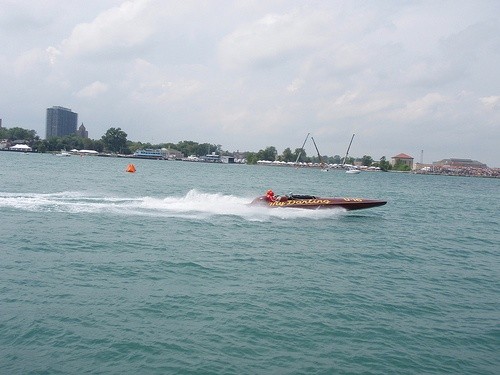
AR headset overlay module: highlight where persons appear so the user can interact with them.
[267,190,276,202]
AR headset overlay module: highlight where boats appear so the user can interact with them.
[131,147,171,161]
[246,193,387,213]
[345,168,362,175]
